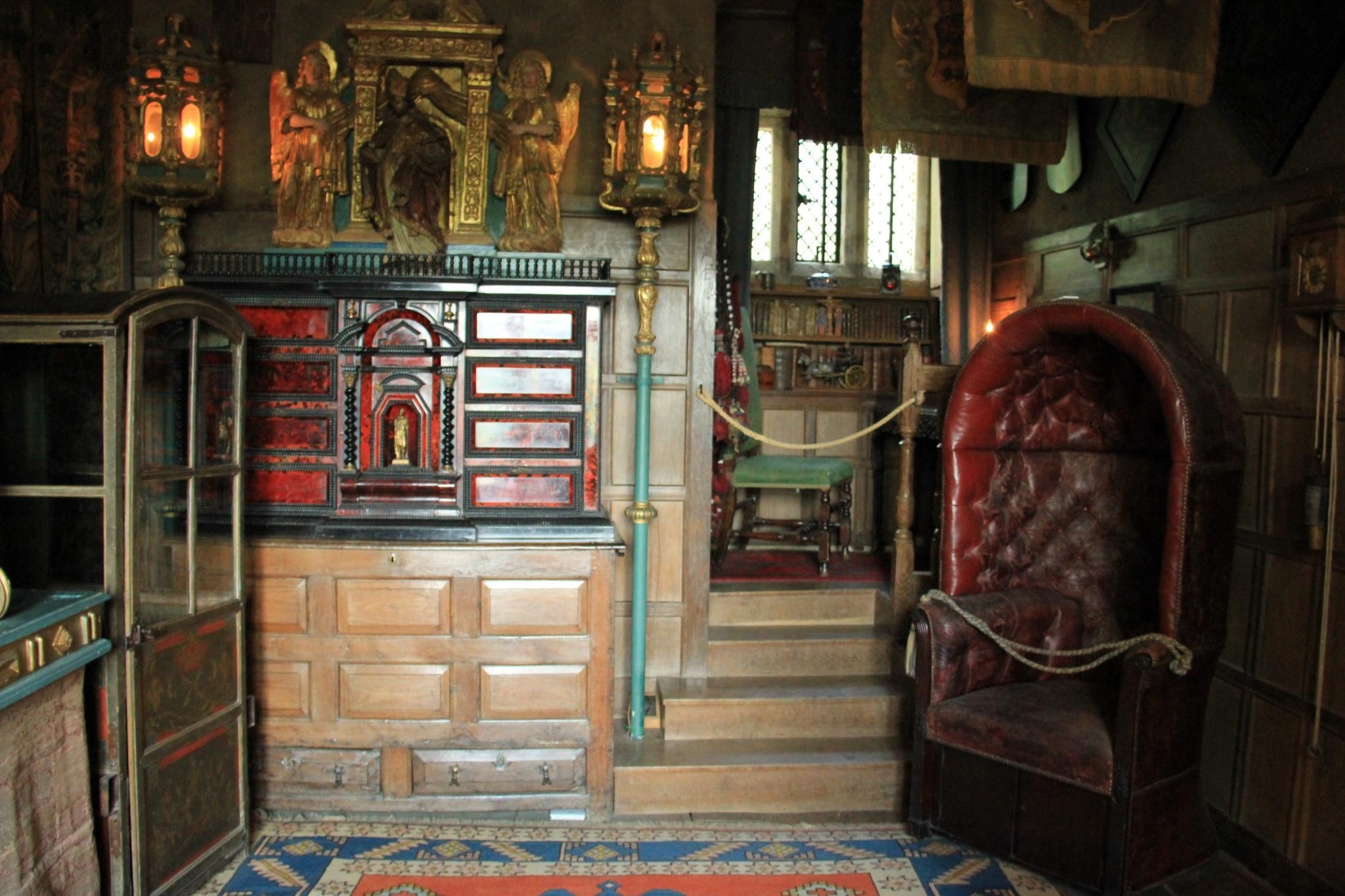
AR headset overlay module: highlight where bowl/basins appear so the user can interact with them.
[808,269,837,286]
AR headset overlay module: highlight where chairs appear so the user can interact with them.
[715,307,854,577]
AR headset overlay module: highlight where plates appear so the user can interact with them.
[806,286,839,293]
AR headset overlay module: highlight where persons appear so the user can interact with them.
[391,408,411,464]
[269,50,349,249]
[215,404,234,454]
[358,68,452,275]
[493,58,563,252]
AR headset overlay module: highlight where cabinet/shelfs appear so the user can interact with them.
[748,284,941,412]
[0,287,255,896]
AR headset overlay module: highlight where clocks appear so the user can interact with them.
[1277,199,1345,321]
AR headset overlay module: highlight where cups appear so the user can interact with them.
[754,270,776,292]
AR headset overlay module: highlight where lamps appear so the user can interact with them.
[599,22,710,221]
[122,9,225,288]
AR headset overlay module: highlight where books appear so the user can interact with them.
[750,298,930,340]
[752,342,936,392]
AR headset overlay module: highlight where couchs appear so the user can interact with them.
[906,300,1238,896]
